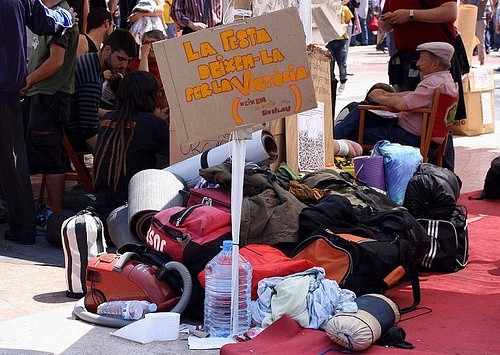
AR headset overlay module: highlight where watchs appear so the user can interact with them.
[410,10,414,21]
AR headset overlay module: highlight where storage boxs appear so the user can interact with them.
[454,65,495,136]
[264,44,334,176]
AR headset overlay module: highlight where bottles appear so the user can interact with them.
[97,300,157,320]
[380,265,406,295]
[203,240,252,338]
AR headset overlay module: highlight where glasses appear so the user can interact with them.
[104,20,117,30]
[142,41,155,45]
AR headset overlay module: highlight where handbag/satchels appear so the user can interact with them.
[46,139,470,350]
[368,14,379,31]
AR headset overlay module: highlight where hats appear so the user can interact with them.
[415,42,455,61]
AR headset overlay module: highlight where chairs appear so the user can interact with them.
[38,130,93,204]
[358,83,459,166]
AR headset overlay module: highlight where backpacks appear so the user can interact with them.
[481,156,500,199]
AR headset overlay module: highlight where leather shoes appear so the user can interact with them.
[4,230,36,245]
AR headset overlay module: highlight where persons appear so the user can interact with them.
[0,0,79,246]
[94,70,169,202]
[460,0,500,66]
[68,0,177,150]
[19,0,79,235]
[331,0,395,83]
[128,29,168,111]
[379,0,470,174]
[171,0,220,35]
[333,42,457,145]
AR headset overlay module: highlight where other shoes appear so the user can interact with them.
[473,51,478,56]
[486,50,490,54]
[339,83,345,92]
[493,47,499,51]
[376,43,384,50]
